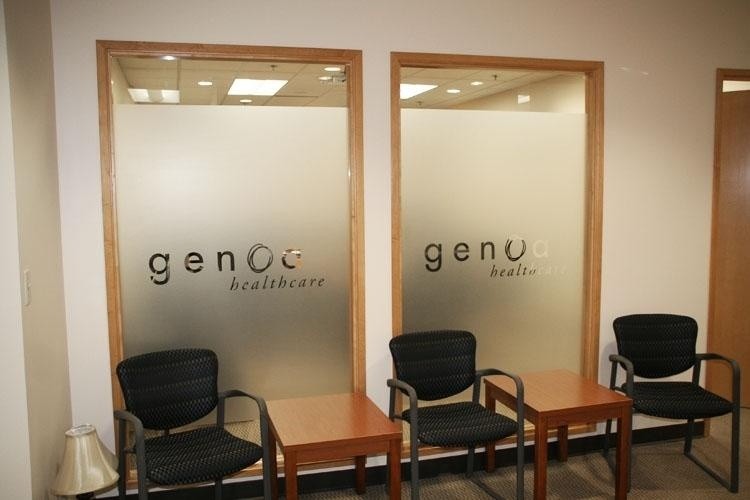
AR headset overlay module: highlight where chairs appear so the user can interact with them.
[386,328,526,500]
[114,345,273,497]
[605,314,742,496]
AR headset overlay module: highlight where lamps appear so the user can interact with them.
[50,424,121,498]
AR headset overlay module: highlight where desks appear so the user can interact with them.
[481,365,632,499]
[264,389,403,499]
